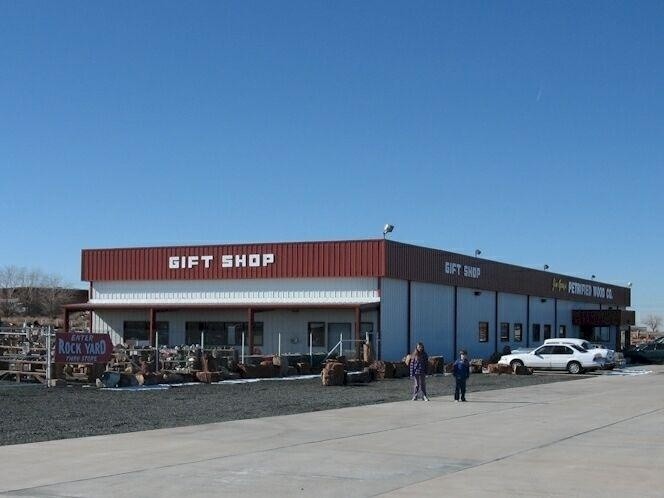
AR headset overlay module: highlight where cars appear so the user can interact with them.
[496,343,603,376]
[510,338,616,371]
[618,336,664,364]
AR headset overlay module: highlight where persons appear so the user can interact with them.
[409,342,429,402]
[451,348,470,403]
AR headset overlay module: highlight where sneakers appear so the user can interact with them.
[423,395,429,401]
[412,395,417,400]
[455,399,465,402]
